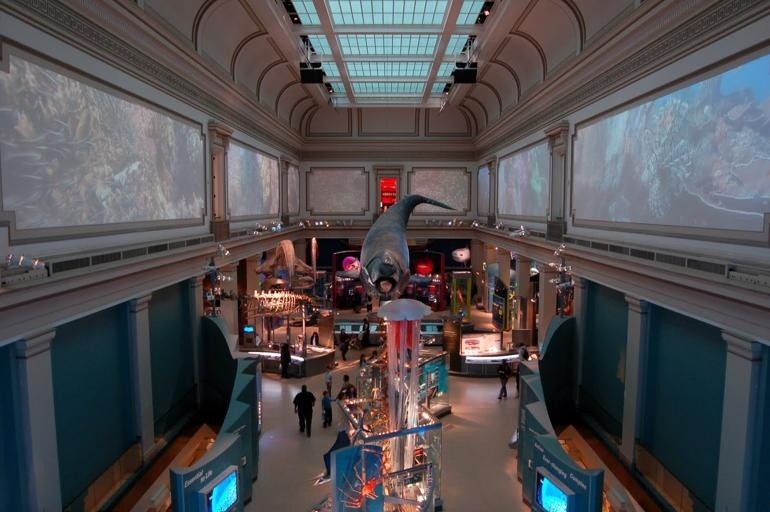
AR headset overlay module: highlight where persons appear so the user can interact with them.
[325,366,332,396]
[338,329,350,360]
[280,343,292,379]
[359,353,367,368]
[320,391,336,428]
[326,285,332,298]
[292,384,316,437]
[370,351,377,360]
[351,291,372,313]
[362,318,371,347]
[310,332,319,345]
[335,374,357,410]
[515,343,528,398]
[472,283,478,306]
[497,357,511,399]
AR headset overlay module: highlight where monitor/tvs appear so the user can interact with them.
[243,326,256,333]
[534,465,576,512]
[197,464,241,512]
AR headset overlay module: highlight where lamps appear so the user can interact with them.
[453,41,478,84]
[7,254,45,270]
[300,41,323,83]
[448,217,575,293]
[203,218,329,280]
